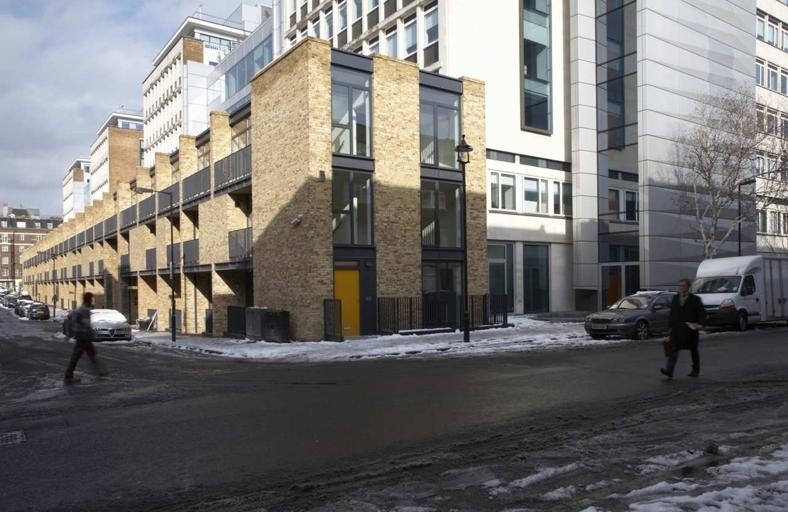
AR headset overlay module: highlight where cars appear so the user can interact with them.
[584,290,679,340]
[1,285,51,320]
[63,308,131,341]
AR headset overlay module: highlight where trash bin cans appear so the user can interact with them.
[246,307,268,340]
[264,311,289,342]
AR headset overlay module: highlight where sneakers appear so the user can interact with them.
[64,378,81,386]
[688,369,700,377]
[660,367,673,378]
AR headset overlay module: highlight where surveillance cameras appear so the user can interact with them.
[290,215,303,225]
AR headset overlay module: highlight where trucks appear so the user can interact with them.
[691,254,788,333]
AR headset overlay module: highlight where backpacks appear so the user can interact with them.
[63,311,80,339]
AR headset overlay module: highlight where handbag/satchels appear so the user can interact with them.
[663,341,670,358]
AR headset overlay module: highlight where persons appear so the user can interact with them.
[62,292,108,384]
[661,278,706,377]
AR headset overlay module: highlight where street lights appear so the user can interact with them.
[10,262,22,297]
[454,135,475,343]
[134,186,176,341]
[36,250,58,317]
[738,177,758,256]
